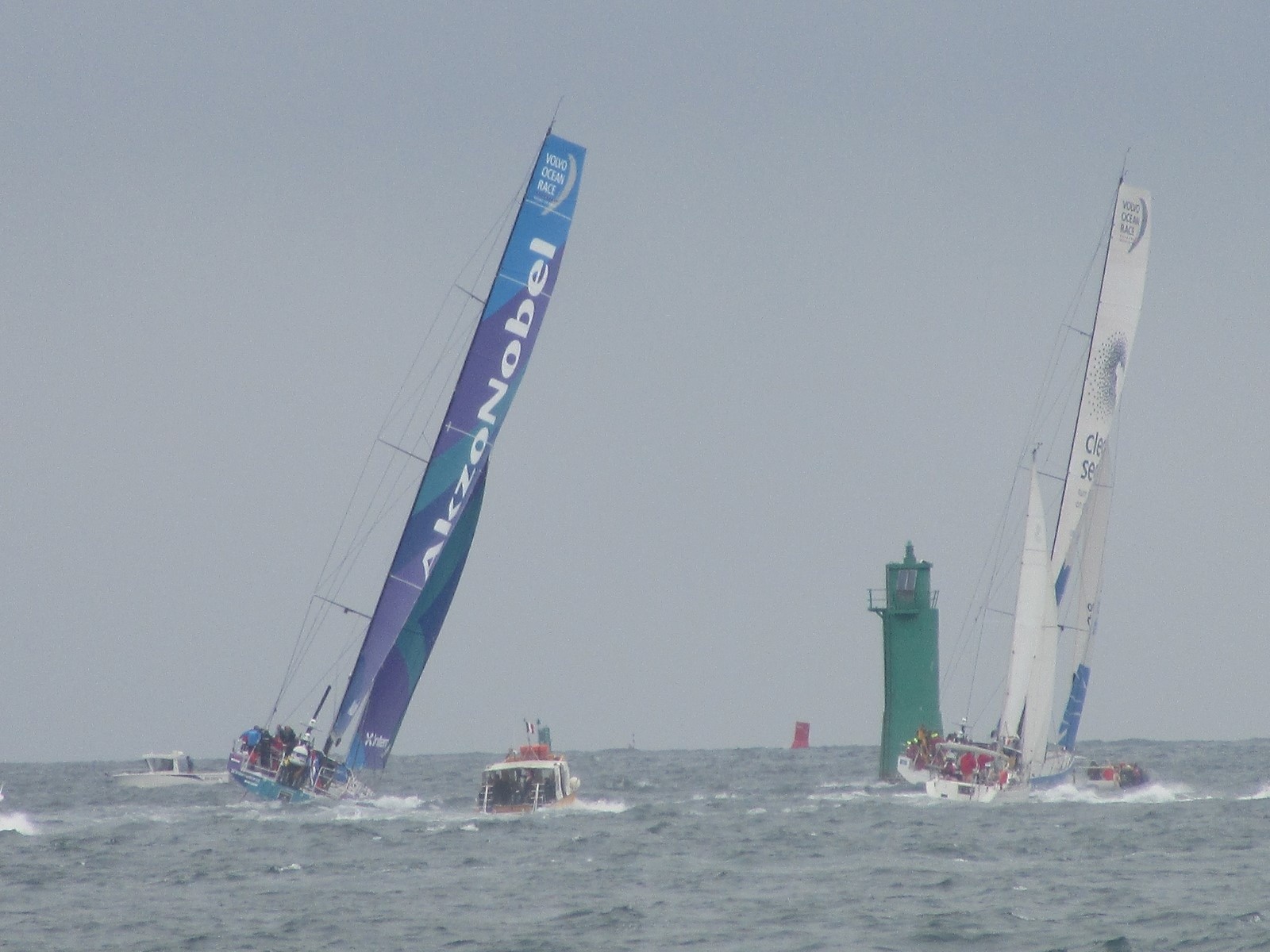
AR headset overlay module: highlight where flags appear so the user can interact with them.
[525,722,535,734]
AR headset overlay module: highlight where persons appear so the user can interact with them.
[237,725,308,789]
[906,724,993,784]
[490,769,533,806]
[1088,759,1145,788]
[185,756,194,774]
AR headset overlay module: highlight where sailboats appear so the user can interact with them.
[227,93,587,804]
[896,148,1151,792]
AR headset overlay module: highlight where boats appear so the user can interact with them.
[109,750,230,791]
[473,718,581,814]
[926,772,1018,804]
